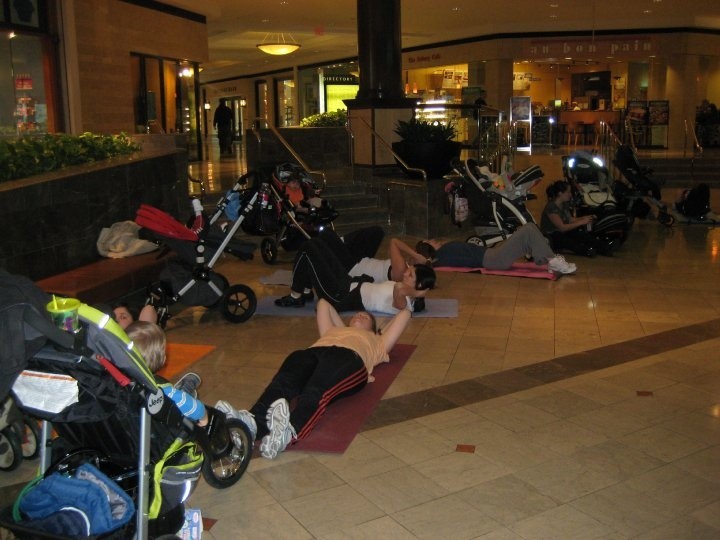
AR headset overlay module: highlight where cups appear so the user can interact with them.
[47,294,83,334]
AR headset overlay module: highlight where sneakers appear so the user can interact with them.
[548,258,576,274]
[260,397,294,458]
[217,400,257,444]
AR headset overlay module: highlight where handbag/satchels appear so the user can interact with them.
[447,193,468,222]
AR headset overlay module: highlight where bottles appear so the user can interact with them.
[257,182,271,210]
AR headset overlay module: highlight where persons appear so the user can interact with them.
[473,90,488,120]
[273,235,437,316]
[111,305,159,331]
[416,221,577,274]
[676,183,711,220]
[696,100,719,125]
[124,321,210,432]
[212,97,234,156]
[317,227,433,286]
[540,179,596,256]
[214,298,411,459]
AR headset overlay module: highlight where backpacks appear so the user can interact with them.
[675,183,712,219]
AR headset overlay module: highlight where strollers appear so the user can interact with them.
[244,162,340,262]
[608,144,675,231]
[1,274,256,539]
[563,152,635,263]
[132,171,281,333]
[454,160,543,257]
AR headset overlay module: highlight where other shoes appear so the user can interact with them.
[174,374,202,397]
[274,291,314,307]
